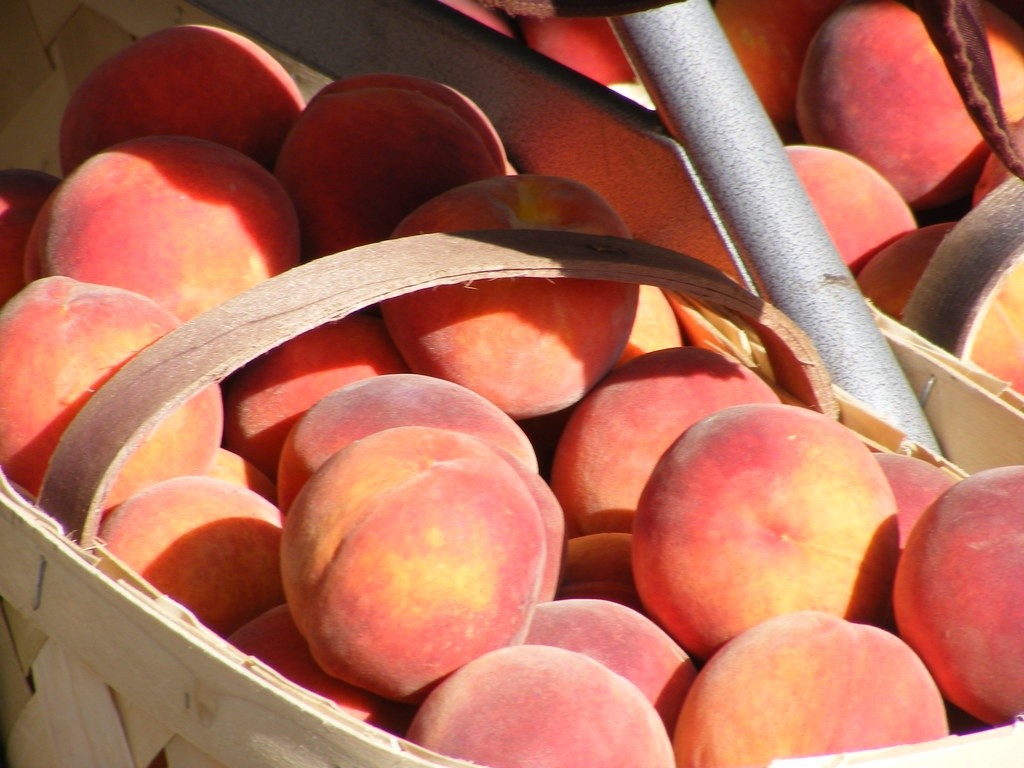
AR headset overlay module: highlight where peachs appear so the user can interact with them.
[0,0,1024,768]
